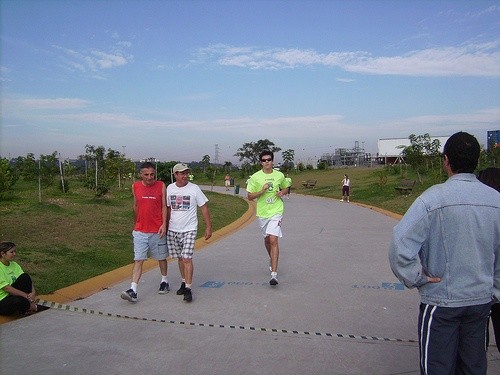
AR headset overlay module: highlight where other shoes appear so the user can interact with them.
[183,288,192,301]
[345,200,349,202]
[34,304,50,313]
[177,282,185,295]
[340,199,343,201]
[269,272,278,286]
[269,259,272,271]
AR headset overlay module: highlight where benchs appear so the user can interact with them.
[302,178,317,189]
[394,179,415,193]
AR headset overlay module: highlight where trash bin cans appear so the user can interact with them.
[234,184,240,196]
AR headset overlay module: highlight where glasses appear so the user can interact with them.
[262,158,272,162]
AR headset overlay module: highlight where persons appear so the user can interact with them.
[247,150,289,286]
[121,162,170,303]
[167,163,212,302]
[246,175,251,199]
[283,173,292,199]
[0,241,37,316]
[188,171,194,183]
[388,131,500,375]
[224,173,232,191]
[341,173,351,203]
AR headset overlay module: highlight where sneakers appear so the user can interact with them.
[120,289,137,302]
[158,281,169,293]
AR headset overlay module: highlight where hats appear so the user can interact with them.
[173,163,192,173]
